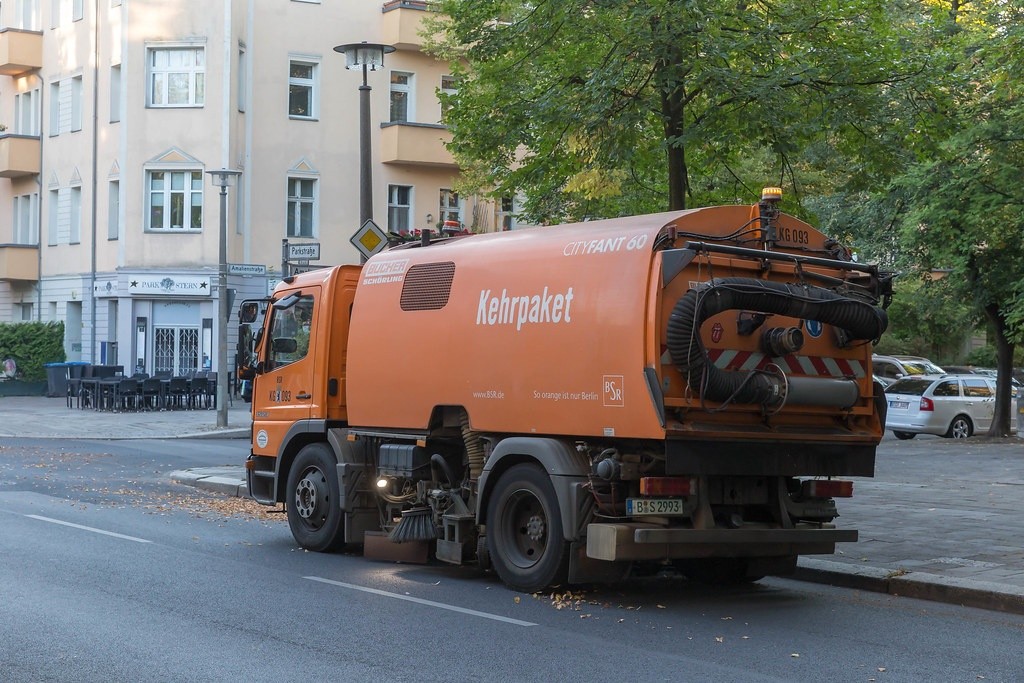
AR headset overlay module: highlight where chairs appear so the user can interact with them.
[67,366,233,413]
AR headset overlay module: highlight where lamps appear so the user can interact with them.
[426,214,432,225]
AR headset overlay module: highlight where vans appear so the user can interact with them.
[884,374,1024,440]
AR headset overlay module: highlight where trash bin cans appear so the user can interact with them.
[43,360,90,397]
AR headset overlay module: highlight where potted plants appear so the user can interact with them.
[0,124,9,134]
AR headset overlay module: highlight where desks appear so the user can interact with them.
[81,380,102,411]
[99,381,192,413]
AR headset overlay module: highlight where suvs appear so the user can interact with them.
[872,355,947,395]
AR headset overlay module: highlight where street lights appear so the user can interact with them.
[205,167,243,427]
[333,41,396,264]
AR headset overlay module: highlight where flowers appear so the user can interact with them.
[390,229,477,240]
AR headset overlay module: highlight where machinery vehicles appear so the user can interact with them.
[237,187,899,596]
